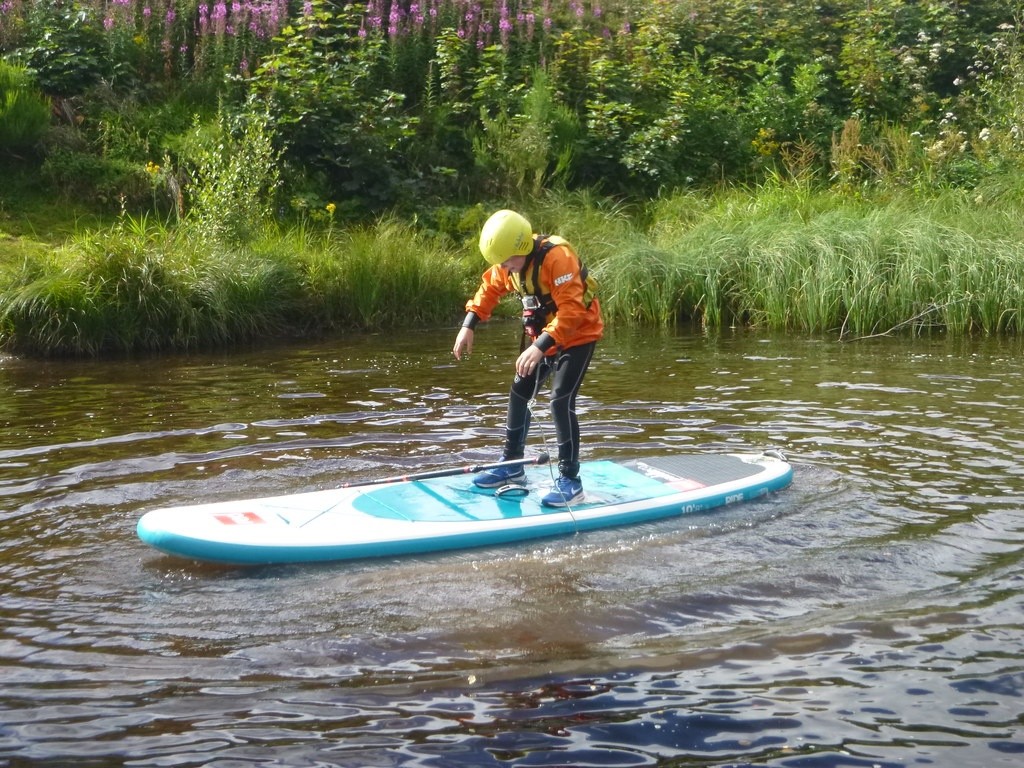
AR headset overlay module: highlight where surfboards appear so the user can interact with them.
[138,452,791,565]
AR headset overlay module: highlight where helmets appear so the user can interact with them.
[478,210,534,265]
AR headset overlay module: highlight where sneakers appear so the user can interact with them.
[541,475,585,507]
[472,457,527,488]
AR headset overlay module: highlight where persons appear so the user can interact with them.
[453,209,604,509]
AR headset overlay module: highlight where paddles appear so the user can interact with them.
[336,452,550,488]
